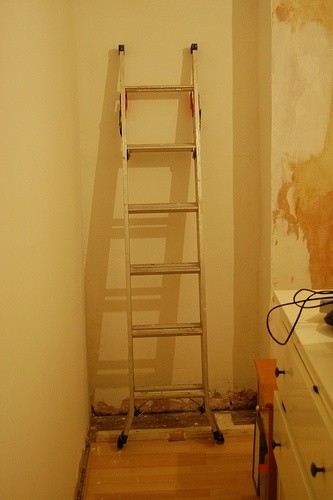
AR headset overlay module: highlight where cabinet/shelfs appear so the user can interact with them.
[270,289,333,500]
[251,356,279,500]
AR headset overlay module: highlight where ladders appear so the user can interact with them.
[117,42,224,447]
